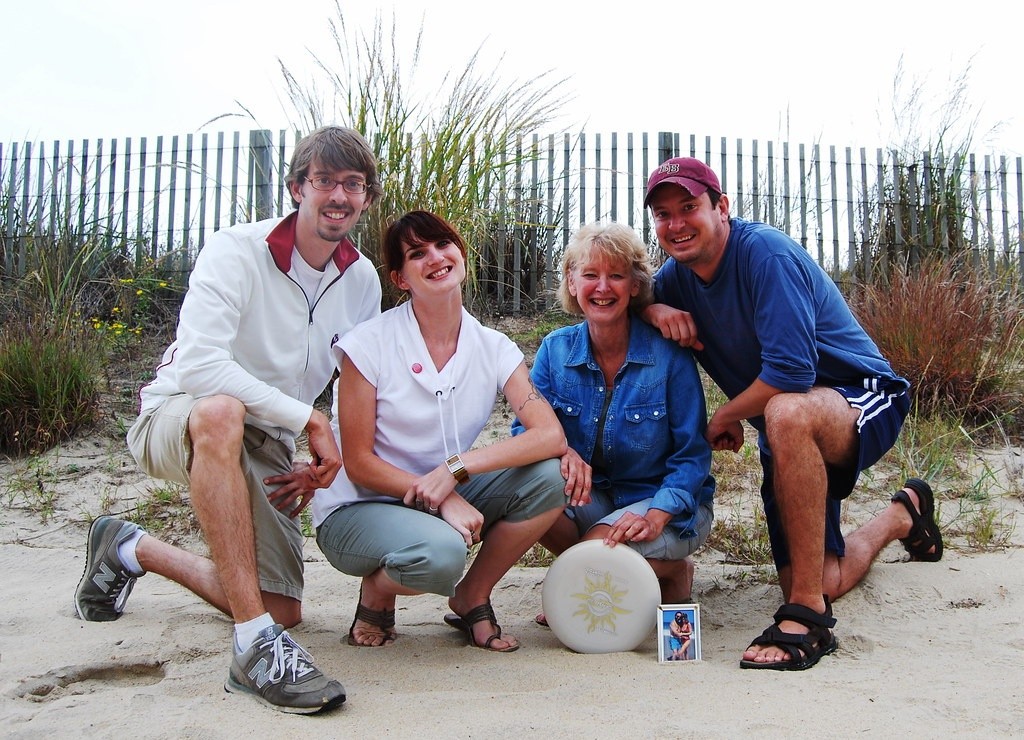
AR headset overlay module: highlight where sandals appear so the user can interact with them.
[740,594,838,671]
[890,478,943,563]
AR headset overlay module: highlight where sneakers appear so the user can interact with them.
[74,515,146,622]
[225,624,347,715]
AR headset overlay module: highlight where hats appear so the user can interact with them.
[643,156,721,210]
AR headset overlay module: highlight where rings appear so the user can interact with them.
[429,507,438,512]
[471,530,475,537]
[415,499,423,506]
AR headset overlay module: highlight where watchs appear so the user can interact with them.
[445,454,470,486]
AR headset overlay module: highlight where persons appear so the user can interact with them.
[75,125,384,714]
[311,211,572,653]
[628,158,944,670]
[511,221,716,627]
[669,611,692,661]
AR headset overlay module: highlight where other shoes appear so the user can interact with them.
[535,611,549,626]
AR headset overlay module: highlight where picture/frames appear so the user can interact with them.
[657,604,701,664]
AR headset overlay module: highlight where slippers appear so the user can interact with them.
[347,583,396,646]
[444,603,519,652]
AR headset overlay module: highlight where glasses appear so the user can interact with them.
[302,174,372,195]
